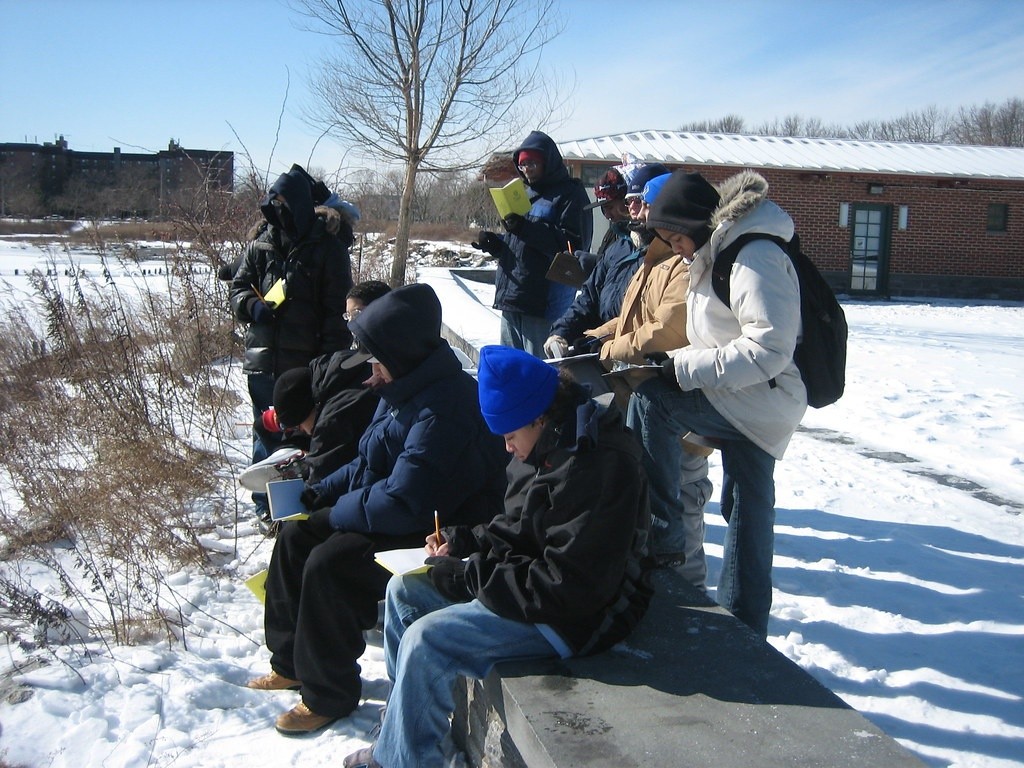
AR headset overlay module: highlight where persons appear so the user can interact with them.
[623,167,806,643]
[568,164,714,597]
[343,343,651,768]
[540,162,669,411]
[472,128,596,361]
[223,164,391,537]
[246,284,507,735]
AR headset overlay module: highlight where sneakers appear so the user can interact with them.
[275,702,338,735]
[248,670,303,689]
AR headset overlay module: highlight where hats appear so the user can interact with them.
[273,367,316,427]
[625,163,670,198]
[518,150,544,165]
[477,345,557,434]
[641,173,672,205]
[340,340,373,369]
[582,169,626,210]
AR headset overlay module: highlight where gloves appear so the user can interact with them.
[308,506,332,539]
[501,213,525,236]
[542,332,602,359]
[253,300,276,322]
[301,489,325,511]
[643,352,668,366]
[574,249,596,270]
[471,231,498,254]
[658,359,673,379]
[425,556,473,605]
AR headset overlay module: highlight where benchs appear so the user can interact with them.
[447,568,930,768]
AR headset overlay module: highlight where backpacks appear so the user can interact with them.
[712,233,847,409]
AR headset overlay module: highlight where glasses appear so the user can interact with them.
[270,198,289,209]
[294,424,300,432]
[518,162,538,171]
[624,198,643,207]
[342,308,362,321]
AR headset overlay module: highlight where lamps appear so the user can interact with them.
[838,200,849,231]
[897,202,910,232]
[868,184,885,195]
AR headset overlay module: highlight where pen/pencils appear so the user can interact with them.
[568,241,572,254]
[435,511,441,546]
[585,332,614,345]
[251,283,275,318]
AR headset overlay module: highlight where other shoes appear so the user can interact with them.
[646,550,688,569]
[342,746,382,768]
[257,512,274,536]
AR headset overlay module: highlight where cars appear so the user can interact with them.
[43,214,64,220]
[79,215,147,223]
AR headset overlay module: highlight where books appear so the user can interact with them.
[264,278,288,309]
[488,179,533,219]
[373,544,473,579]
[245,568,268,606]
[264,480,311,521]
